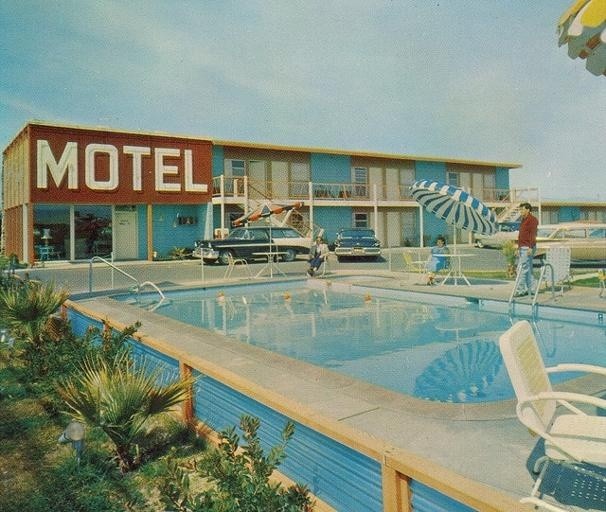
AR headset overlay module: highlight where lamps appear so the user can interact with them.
[40,228,52,246]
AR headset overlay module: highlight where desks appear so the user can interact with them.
[251,251,289,279]
[36,247,54,260]
[431,251,475,291]
[433,309,480,332]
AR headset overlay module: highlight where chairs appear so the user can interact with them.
[306,241,329,283]
[493,319,605,511]
[539,245,571,296]
[400,247,433,289]
[224,249,254,281]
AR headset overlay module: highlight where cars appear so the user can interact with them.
[334,227,381,260]
[473,221,520,250]
[527,222,606,267]
[193,226,311,265]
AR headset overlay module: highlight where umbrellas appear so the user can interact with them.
[408,178,498,286]
[232,202,304,279]
[412,331,505,402]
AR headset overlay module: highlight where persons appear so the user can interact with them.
[216,229,221,238]
[307,236,329,276]
[514,202,539,297]
[428,237,448,283]
